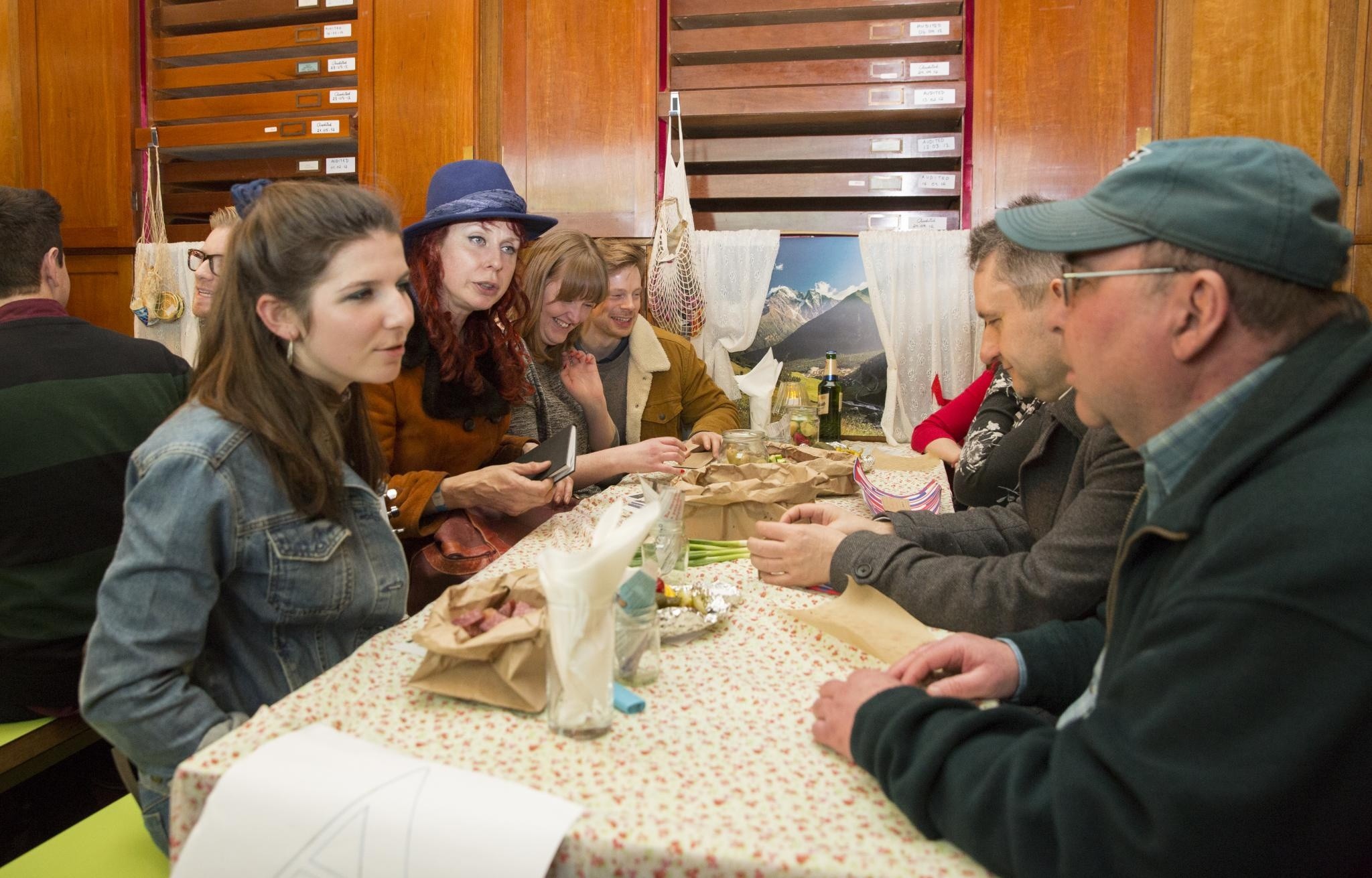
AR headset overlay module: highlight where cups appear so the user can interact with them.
[748,397,772,431]
[543,592,618,740]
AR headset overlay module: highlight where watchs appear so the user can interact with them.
[432,486,445,512]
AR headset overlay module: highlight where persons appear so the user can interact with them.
[186,205,243,318]
[0,189,197,725]
[576,237,738,489]
[516,230,690,499]
[77,177,416,862]
[746,195,1144,638]
[817,136,1372,878]
[358,160,574,618]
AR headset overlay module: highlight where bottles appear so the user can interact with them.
[615,602,661,685]
[641,519,689,584]
[785,406,819,447]
[818,350,842,443]
[718,429,769,465]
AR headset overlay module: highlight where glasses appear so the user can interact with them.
[1061,262,1233,307]
[188,247,226,279]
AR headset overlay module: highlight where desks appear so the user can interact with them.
[167,433,1006,878]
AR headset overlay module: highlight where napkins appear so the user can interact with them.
[525,493,664,732]
[732,346,784,431]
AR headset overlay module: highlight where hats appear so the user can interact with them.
[401,160,558,249]
[995,136,1353,290]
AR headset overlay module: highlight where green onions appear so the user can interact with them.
[628,539,753,566]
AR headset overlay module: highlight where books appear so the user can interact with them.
[514,424,577,484]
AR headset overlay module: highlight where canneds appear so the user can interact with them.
[784,406,819,447]
[718,428,769,464]
